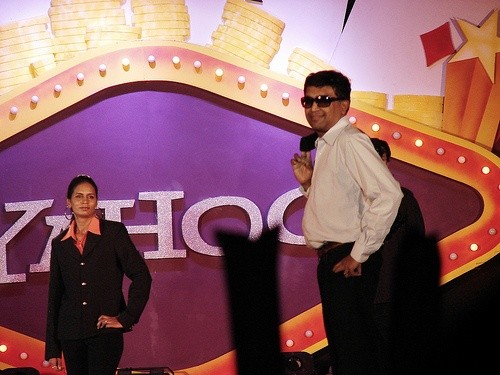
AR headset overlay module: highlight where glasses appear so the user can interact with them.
[301,95,344,108]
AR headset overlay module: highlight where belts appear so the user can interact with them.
[317,242,344,256]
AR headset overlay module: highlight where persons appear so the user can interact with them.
[44,175,151,375]
[290,69,404,360]
[370,137,426,311]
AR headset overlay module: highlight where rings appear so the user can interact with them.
[54,365,57,367]
[52,367,54,368]
[104,320,108,324]
